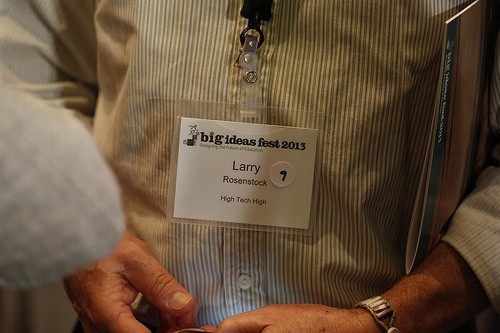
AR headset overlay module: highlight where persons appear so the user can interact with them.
[0,1,499,333]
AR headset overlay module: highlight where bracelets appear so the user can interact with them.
[353,295,400,333]
